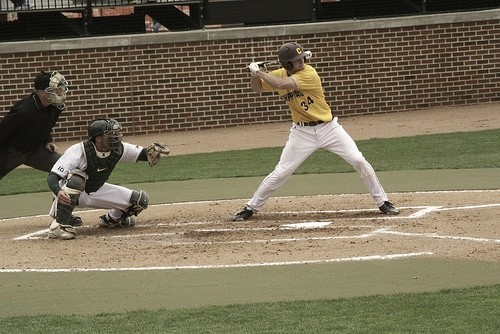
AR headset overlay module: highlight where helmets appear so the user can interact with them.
[278,41,308,70]
[34,70,67,90]
[86,118,119,141]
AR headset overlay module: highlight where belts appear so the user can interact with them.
[296,120,324,126]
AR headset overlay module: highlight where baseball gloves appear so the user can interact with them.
[146,141,170,167]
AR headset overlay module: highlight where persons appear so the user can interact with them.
[233,43,400,222]
[0,70,84,226]
[47,118,171,238]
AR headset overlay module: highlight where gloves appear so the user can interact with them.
[248,62,268,74]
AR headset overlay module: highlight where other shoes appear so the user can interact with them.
[69,214,83,225]
[48,223,77,240]
[233,207,253,221]
[98,212,136,228]
[380,201,399,215]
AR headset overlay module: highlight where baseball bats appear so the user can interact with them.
[246,51,312,73]
